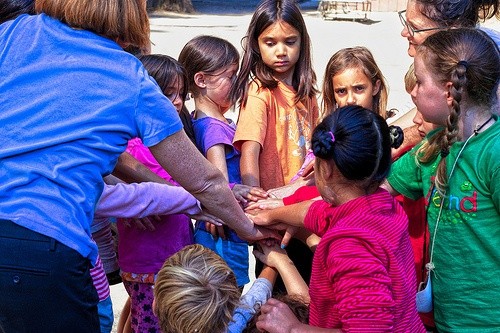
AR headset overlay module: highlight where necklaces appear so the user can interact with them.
[425,114,498,270]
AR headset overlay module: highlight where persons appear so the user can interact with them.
[244,105,429,333]
[152,244,279,333]
[86,0,500,333]
[242,243,311,333]
[265,28,500,333]
[0,0,288,333]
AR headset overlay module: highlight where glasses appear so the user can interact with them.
[398,10,442,37]
